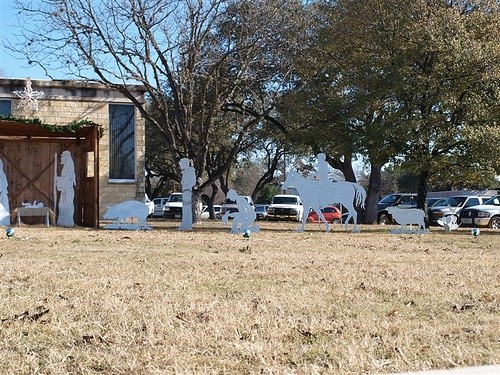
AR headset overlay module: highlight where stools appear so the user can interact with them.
[11,207,55,227]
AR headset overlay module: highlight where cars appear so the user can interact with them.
[163,192,183,219]
[377,193,418,225]
[256,206,269,220]
[306,206,342,224]
[148,198,169,219]
[201,206,222,219]
[459,195,500,229]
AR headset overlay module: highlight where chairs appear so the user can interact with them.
[173,197,179,202]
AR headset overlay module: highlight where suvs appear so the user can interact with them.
[267,194,304,223]
[428,196,491,226]
